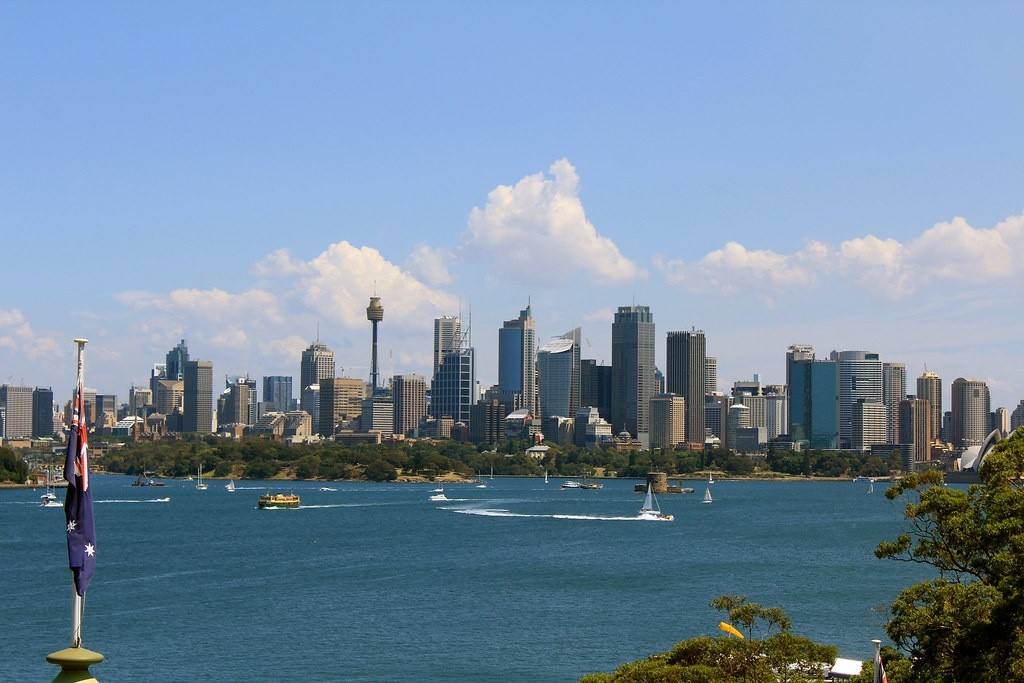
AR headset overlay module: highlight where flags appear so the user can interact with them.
[64,381,97,596]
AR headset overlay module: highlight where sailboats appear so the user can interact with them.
[489,466,493,480]
[702,487,713,503]
[132,460,165,486]
[709,469,714,483]
[41,447,57,500]
[544,470,549,483]
[224,478,235,492]
[869,483,874,493]
[637,482,674,521]
[196,464,208,490]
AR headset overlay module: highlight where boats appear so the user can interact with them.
[428,488,443,493]
[580,483,603,489]
[319,487,337,491]
[157,498,170,501]
[562,481,581,488]
[855,475,874,483]
[180,477,193,482]
[477,485,486,488]
[258,485,301,508]
[428,494,448,501]
[40,498,64,508]
